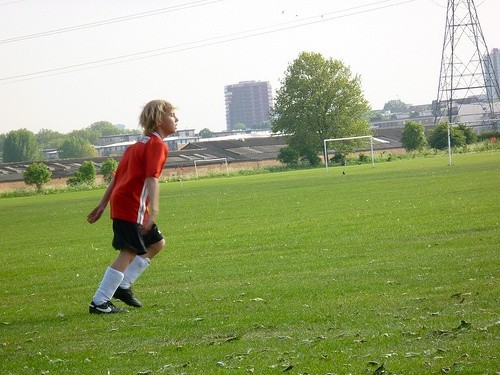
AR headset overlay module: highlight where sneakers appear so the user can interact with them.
[89,302,130,314]
[114,286,142,307]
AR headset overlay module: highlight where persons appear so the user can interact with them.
[87,99,180,314]
